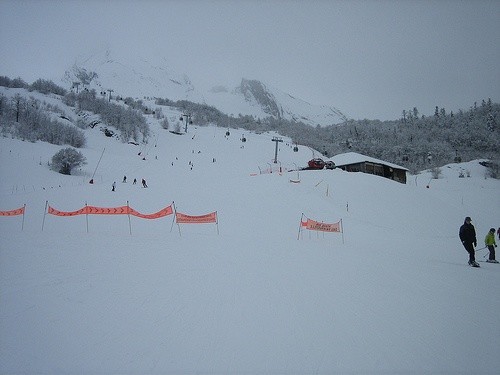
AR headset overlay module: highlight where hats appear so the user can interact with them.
[465,217,472,221]
[490,228,496,232]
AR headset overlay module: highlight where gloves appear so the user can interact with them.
[474,242,477,247]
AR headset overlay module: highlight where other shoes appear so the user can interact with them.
[492,259,499,263]
[489,259,493,262]
[472,261,478,265]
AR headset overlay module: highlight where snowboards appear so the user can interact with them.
[468,260,481,267]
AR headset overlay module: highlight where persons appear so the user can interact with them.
[141,178,148,188]
[132,178,136,184]
[122,176,126,182]
[484,228,497,263]
[225,130,231,140]
[239,133,246,150]
[459,217,480,267]
[497,228,500,240]
[112,182,117,191]
